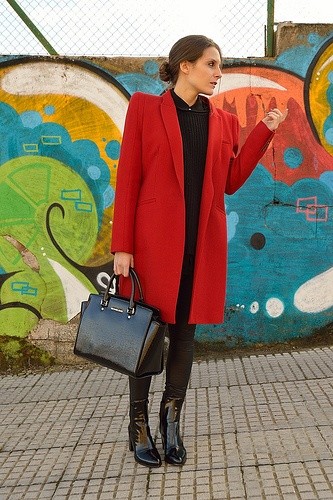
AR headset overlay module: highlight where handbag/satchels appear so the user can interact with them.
[73,267,166,379]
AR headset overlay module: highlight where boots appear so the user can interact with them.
[128,398,162,468]
[159,392,187,466]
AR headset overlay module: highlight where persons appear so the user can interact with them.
[110,35,290,467]
